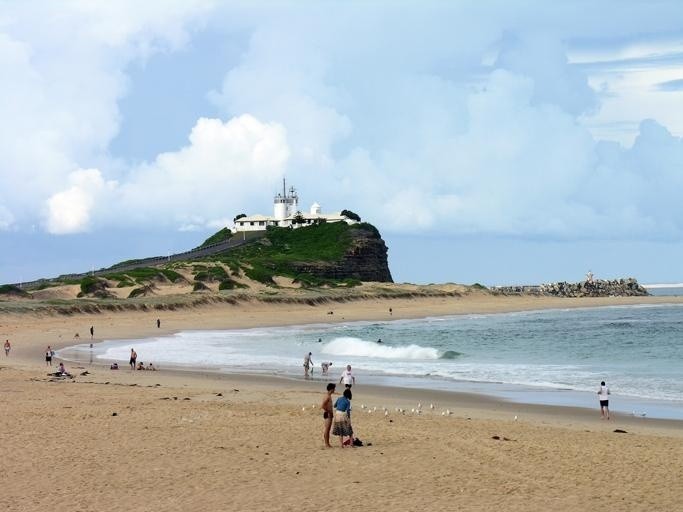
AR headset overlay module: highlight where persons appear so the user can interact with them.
[90,326,95,338]
[137,361,145,370]
[111,362,119,369]
[597,381,611,420]
[333,389,355,448]
[45,346,52,366]
[4,340,11,357]
[321,361,333,375]
[156,318,161,328]
[339,365,355,389]
[129,348,137,370]
[321,382,336,448]
[389,307,393,316]
[146,363,160,371]
[47,362,65,376]
[302,351,314,377]
